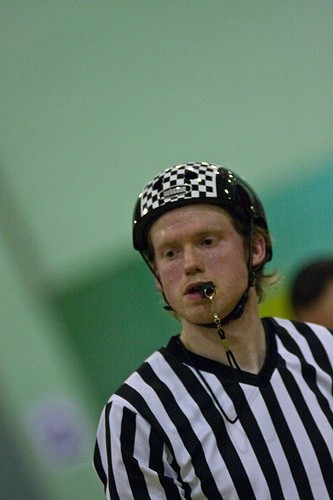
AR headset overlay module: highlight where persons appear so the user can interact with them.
[91,160,333,500]
[287,254,332,335]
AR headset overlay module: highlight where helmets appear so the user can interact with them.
[131,161,275,263]
